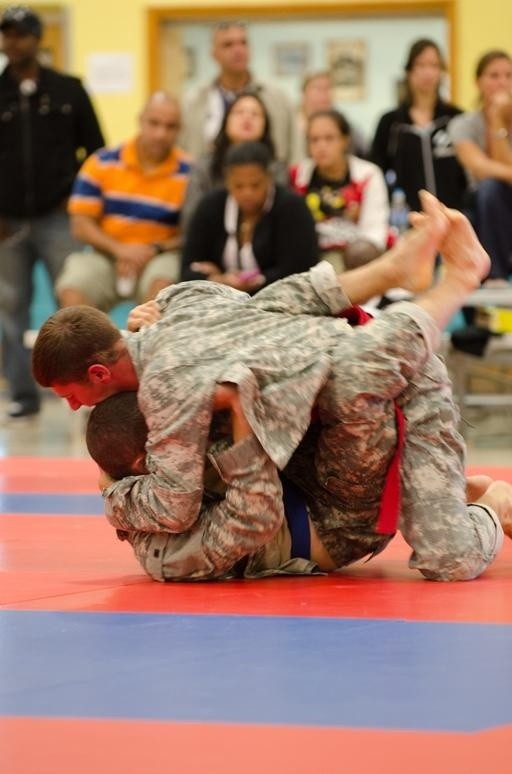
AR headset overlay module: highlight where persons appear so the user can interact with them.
[179,23,297,180]
[85,189,492,582]
[282,70,371,176]
[176,96,295,249]
[286,112,390,263]
[53,93,209,315]
[0,6,109,415]
[451,50,512,333]
[369,40,469,219]
[180,140,322,305]
[33,279,512,582]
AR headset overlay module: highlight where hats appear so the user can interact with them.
[1,8,46,37]
